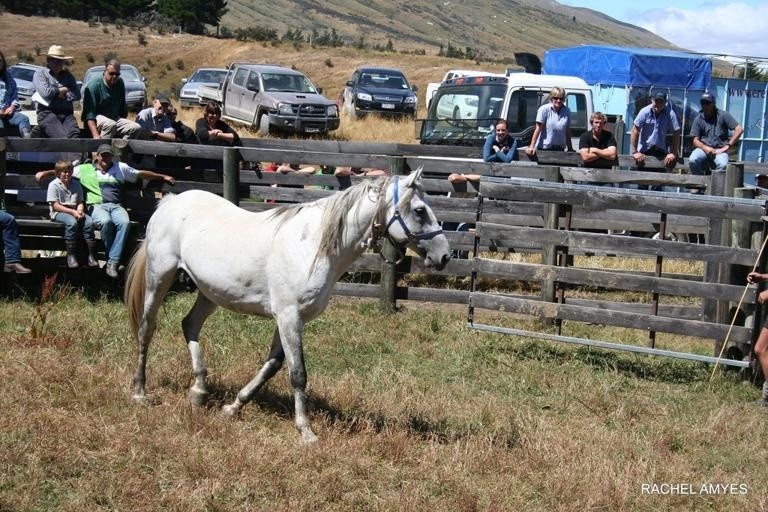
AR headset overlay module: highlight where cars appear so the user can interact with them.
[177,69,238,111]
[73,62,151,114]
[344,67,419,124]
[3,63,58,109]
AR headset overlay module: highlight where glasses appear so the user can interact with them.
[109,72,121,76]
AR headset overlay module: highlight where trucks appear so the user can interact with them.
[417,46,768,254]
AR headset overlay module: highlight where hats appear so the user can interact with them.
[39,45,75,60]
[97,144,114,157]
[156,95,169,104]
[654,91,666,102]
[700,93,714,102]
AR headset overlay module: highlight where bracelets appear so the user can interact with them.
[725,143,731,148]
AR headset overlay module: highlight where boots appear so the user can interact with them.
[66,240,79,268]
[86,241,98,266]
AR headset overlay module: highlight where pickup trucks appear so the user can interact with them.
[197,62,339,139]
[425,66,514,131]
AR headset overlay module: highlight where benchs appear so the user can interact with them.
[0,214,140,252]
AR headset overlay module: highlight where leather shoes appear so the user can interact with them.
[4,263,32,273]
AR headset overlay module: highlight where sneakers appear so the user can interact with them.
[106,264,125,277]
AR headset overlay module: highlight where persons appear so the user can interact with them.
[578,112,617,187]
[689,92,744,195]
[483,119,519,180]
[526,86,576,183]
[0,43,482,278]
[630,90,680,192]
[746,215,768,401]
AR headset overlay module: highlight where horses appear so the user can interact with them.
[124,163,452,446]
